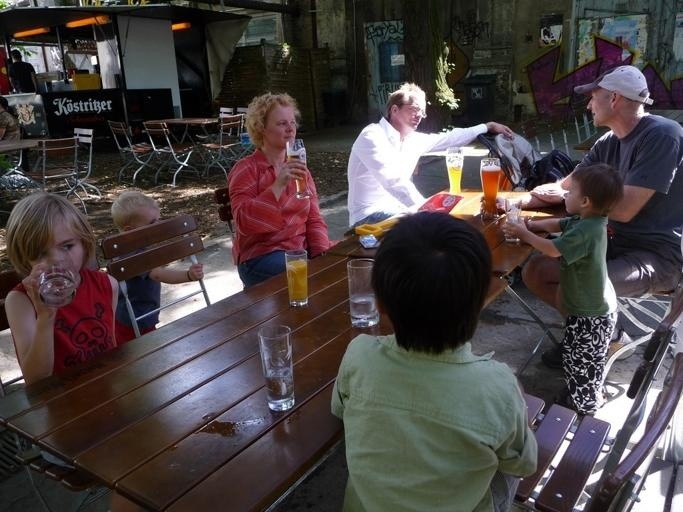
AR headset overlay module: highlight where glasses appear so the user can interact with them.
[402,104,427,118]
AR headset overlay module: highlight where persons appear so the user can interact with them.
[348,82,515,238]
[4,193,119,385]
[480,65,683,370]
[502,165,623,426]
[111,191,204,346]
[0,96,21,165]
[8,49,38,93]
[226,92,344,288]
[331,212,538,511]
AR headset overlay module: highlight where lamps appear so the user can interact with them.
[65,15,110,28]
[171,23,188,31]
[14,26,48,40]
[90,55,98,67]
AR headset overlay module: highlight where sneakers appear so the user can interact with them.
[608,329,636,361]
[542,343,563,369]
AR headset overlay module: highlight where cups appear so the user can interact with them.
[346,256,381,331]
[257,323,295,414]
[502,194,523,245]
[478,158,503,224]
[445,144,465,195]
[34,244,79,308]
[284,138,313,202]
[283,248,309,308]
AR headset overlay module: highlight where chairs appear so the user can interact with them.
[108,121,159,184]
[236,107,253,141]
[32,129,102,215]
[103,213,210,338]
[144,122,199,188]
[211,186,239,240]
[0,269,106,512]
[197,107,235,144]
[26,136,80,198]
[515,351,683,512]
[203,115,244,184]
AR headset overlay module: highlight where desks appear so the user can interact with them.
[328,192,566,352]
[148,117,214,188]
[0,254,510,512]
[0,138,63,179]
[4,87,175,152]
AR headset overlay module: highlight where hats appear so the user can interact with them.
[574,65,654,104]
